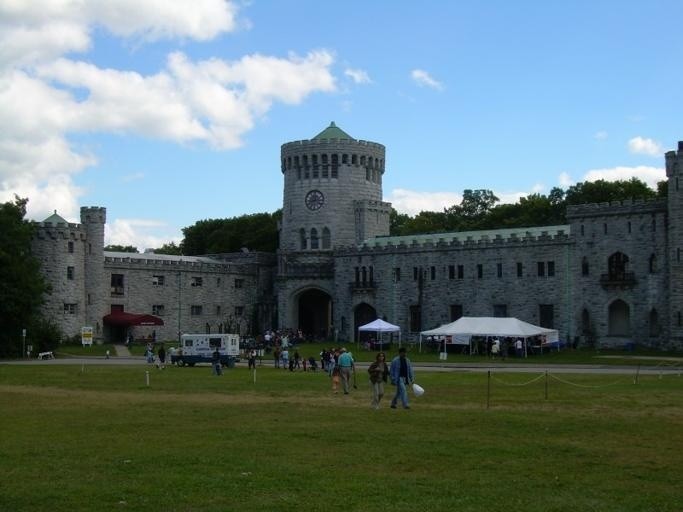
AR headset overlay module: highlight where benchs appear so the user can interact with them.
[37,351,54,360]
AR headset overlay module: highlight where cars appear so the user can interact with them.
[239,328,297,350]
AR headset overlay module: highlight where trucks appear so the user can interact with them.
[171,334,240,368]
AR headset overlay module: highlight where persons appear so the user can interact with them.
[105,349,109,360]
[144,343,184,370]
[211,328,358,394]
[367,352,390,409]
[389,348,414,409]
[491,334,546,361]
[119,332,133,350]
[367,333,375,352]
[431,335,445,355]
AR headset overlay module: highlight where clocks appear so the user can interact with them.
[305,190,324,211]
